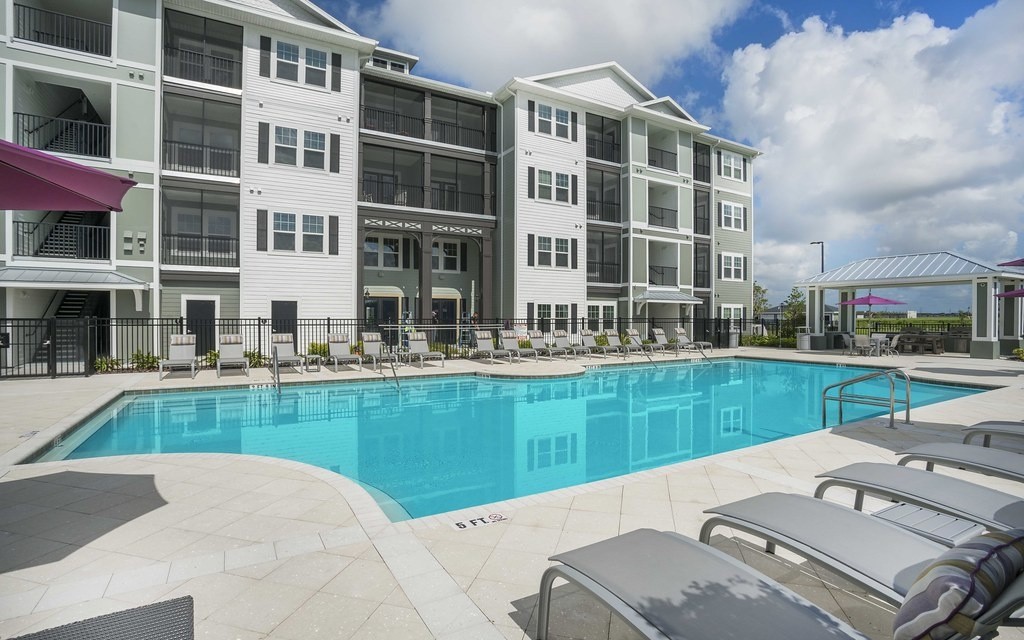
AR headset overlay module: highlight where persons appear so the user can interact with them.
[470,312,480,349]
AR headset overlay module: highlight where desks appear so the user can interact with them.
[395,351,410,365]
[879,499,985,548]
[306,355,321,371]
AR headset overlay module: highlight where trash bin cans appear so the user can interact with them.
[728,326,740,348]
[795,326,812,350]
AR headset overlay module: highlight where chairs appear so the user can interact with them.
[322,334,362,371]
[536,528,866,640]
[159,334,199,380]
[216,336,250,378]
[270,333,304,382]
[469,327,714,364]
[894,442,1024,484]
[960,421,1024,448]
[700,492,1024,640]
[813,463,1024,536]
[408,332,445,369]
[359,333,398,371]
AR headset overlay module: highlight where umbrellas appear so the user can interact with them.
[0,139,139,215]
[996,258,1024,267]
[994,288,1024,297]
[836,294,908,338]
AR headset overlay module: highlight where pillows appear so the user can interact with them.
[892,529,1024,640]
